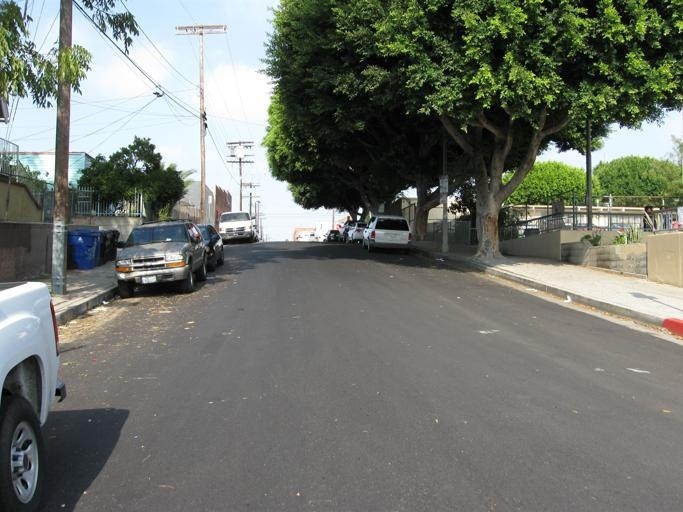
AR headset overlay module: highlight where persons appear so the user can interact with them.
[643,205,657,230]
[344,216,354,226]
[671,217,680,229]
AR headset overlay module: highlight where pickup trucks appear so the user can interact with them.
[0,276,69,509]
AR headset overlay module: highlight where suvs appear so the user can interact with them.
[112,216,206,298]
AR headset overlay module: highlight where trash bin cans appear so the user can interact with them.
[524,228,540,238]
[67,228,120,270]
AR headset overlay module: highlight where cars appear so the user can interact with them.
[294,215,412,253]
[196,223,225,272]
[521,221,632,240]
[218,209,260,243]
